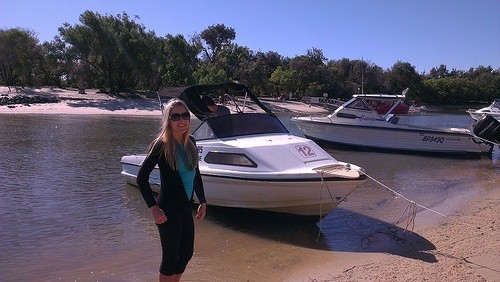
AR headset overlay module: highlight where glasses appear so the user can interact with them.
[168,111,190,120]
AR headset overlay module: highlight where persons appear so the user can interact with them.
[136,97,208,282]
[202,97,231,136]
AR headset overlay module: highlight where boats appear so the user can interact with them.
[120,81,368,224]
[467,101,500,121]
[344,94,411,113]
[288,88,500,156]
